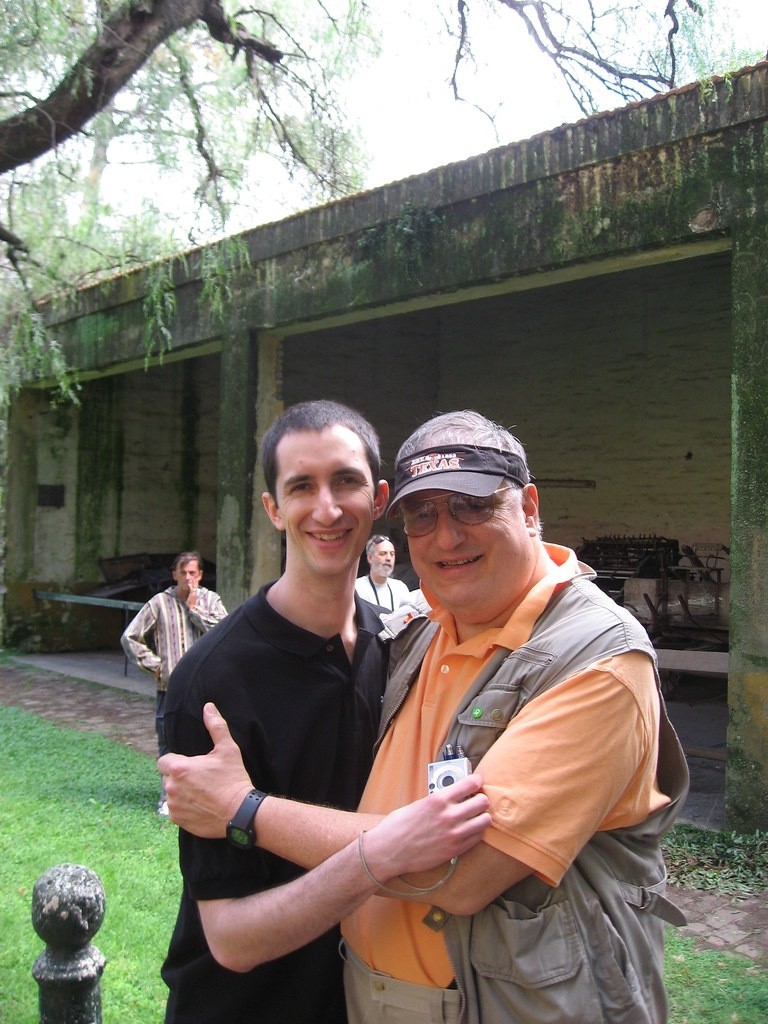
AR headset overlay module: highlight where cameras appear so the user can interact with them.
[427,757,472,796]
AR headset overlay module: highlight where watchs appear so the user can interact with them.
[225,789,268,851]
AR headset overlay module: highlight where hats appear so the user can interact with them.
[385,443,529,520]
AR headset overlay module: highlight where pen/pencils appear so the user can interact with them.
[441,742,465,760]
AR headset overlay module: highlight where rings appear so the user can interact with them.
[194,589,197,592]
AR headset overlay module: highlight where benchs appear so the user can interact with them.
[574,534,729,626]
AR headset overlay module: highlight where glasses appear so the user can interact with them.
[393,486,514,538]
[368,536,389,551]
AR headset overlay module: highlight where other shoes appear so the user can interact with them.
[157,795,170,815]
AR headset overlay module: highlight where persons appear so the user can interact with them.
[162,400,491,1024]
[354,534,409,612]
[155,410,691,1024]
[121,551,228,815]
